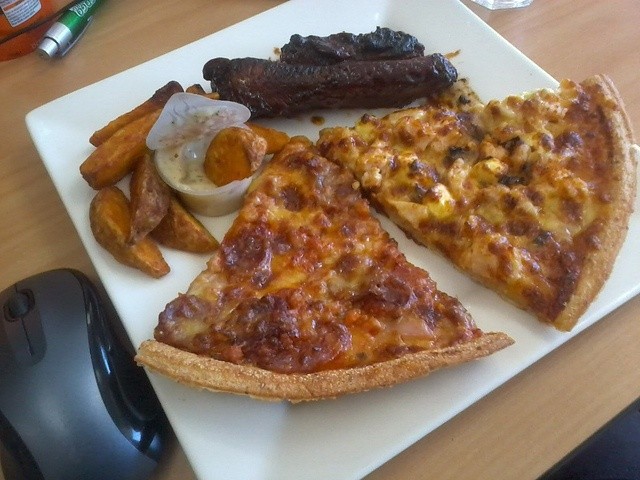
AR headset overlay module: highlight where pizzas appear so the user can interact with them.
[132,133,515,404]
[318,73,640,333]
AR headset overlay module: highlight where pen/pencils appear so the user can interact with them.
[37,0,106,59]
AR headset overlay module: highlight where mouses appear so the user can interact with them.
[0,265,170,479]
